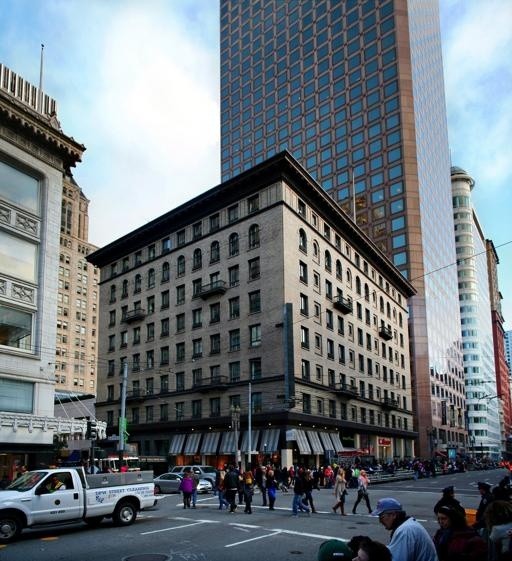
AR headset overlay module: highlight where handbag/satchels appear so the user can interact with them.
[361,485,368,497]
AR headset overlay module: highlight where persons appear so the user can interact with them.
[369,458,511,477]
[215,463,295,514]
[21,465,28,475]
[180,470,199,508]
[293,463,372,516]
[318,497,438,561]
[433,476,512,561]
[45,476,66,492]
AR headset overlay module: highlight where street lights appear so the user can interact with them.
[230,404,242,469]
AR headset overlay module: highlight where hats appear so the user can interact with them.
[442,485,453,493]
[478,480,491,489]
[372,497,402,515]
[318,539,352,560]
[348,535,371,551]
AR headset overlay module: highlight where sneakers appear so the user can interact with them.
[219,502,275,513]
[291,505,318,518]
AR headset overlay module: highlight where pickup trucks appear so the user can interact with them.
[0,467,154,544]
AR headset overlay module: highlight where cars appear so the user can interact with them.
[154,472,212,494]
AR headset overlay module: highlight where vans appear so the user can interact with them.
[170,465,216,487]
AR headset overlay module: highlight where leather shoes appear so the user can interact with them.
[353,511,357,513]
[333,506,336,512]
[341,513,346,515]
